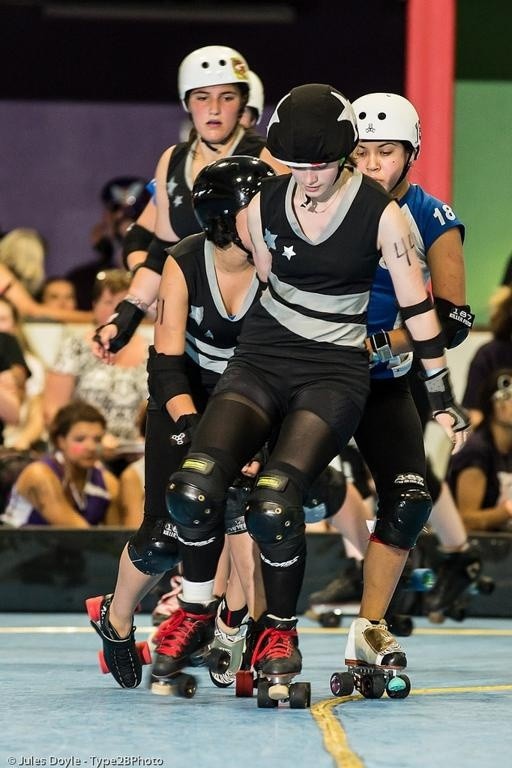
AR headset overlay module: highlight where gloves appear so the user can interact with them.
[93,296,150,355]
[421,369,470,434]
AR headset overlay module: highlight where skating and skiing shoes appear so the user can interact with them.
[237,623,264,697]
[144,574,185,626]
[381,562,436,637]
[210,598,244,688]
[330,616,411,699]
[255,614,311,711]
[316,559,368,627]
[145,592,230,700]
[420,543,496,624]
[86,589,150,689]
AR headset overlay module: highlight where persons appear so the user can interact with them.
[145,81,471,708]
[451,252,512,532]
[87,45,290,687]
[84,155,279,694]
[305,360,495,634]
[226,91,475,699]
[125,70,265,621]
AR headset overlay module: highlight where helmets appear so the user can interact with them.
[350,92,422,156]
[264,84,359,169]
[99,176,152,221]
[191,155,279,252]
[176,45,250,112]
[244,70,264,126]
